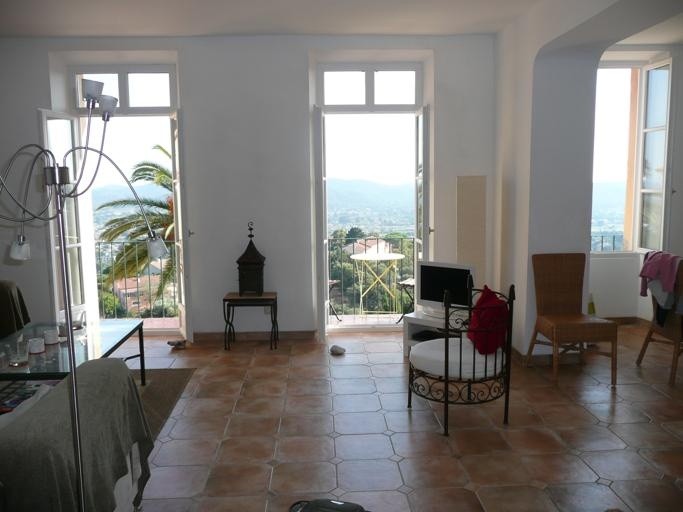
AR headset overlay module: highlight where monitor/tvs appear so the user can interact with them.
[417,260,472,320]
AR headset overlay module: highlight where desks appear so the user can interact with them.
[222,292,280,351]
[350,253,405,321]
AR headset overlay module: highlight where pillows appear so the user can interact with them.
[467,284,509,355]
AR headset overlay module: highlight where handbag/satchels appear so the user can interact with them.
[648,280,674,309]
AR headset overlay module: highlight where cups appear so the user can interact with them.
[43,329,59,341]
[6,339,30,367]
[28,338,45,354]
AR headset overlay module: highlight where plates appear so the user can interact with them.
[42,340,59,345]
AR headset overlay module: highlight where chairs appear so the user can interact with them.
[636,251,682,387]
[523,252,618,388]
[407,273,516,437]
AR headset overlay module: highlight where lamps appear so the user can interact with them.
[0,74,168,512]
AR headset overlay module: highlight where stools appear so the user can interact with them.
[329,279,342,322]
[395,277,414,324]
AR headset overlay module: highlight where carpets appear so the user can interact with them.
[128,368,198,442]
